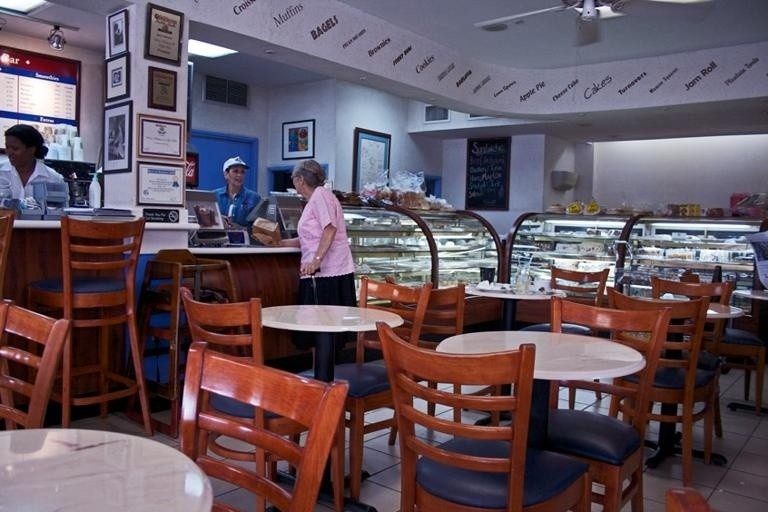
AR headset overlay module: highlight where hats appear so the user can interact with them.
[223,156,249,173]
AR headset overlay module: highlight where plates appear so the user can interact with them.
[475,283,517,293]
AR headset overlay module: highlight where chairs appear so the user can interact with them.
[0,299,71,428]
[180,342,350,512]
[289,275,432,501]
[606,286,718,488]
[26,215,154,437]
[679,268,766,418]
[505,295,674,512]
[375,320,592,511]
[519,265,610,410]
[126,249,247,438]
[650,275,736,439]
[385,275,502,446]
[178,286,345,510]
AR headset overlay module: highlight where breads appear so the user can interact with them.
[251,217,281,246]
[397,191,431,211]
[363,186,391,208]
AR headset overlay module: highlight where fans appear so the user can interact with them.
[474,0,717,45]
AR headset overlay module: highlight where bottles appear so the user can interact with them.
[689,203,700,217]
[679,202,690,217]
[668,202,677,217]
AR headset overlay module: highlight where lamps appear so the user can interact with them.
[47,24,67,51]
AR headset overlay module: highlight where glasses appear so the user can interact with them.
[290,175,299,179]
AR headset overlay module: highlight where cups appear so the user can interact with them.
[478,266,496,285]
[517,267,529,292]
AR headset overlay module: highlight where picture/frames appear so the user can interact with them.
[102,100,133,174]
[282,119,316,160]
[143,2,184,67]
[105,52,130,102]
[352,127,391,193]
[108,9,128,57]
[148,66,177,112]
[136,161,186,207]
[136,112,185,161]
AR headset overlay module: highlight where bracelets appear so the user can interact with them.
[314,256,322,262]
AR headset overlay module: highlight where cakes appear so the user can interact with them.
[586,202,599,214]
[554,243,579,256]
[340,213,365,227]
[546,203,564,215]
[700,251,733,266]
[445,241,456,252]
[373,218,393,227]
[428,200,441,212]
[640,248,663,262]
[451,226,463,232]
[418,237,442,251]
[582,243,603,258]
[398,216,422,229]
[566,202,581,215]
[668,248,690,262]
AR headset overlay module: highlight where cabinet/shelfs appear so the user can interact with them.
[276,199,504,348]
[3,220,123,404]
[189,248,315,360]
[505,212,768,332]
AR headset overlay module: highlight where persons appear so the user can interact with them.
[0,124,70,209]
[213,157,263,243]
[263,160,356,371]
